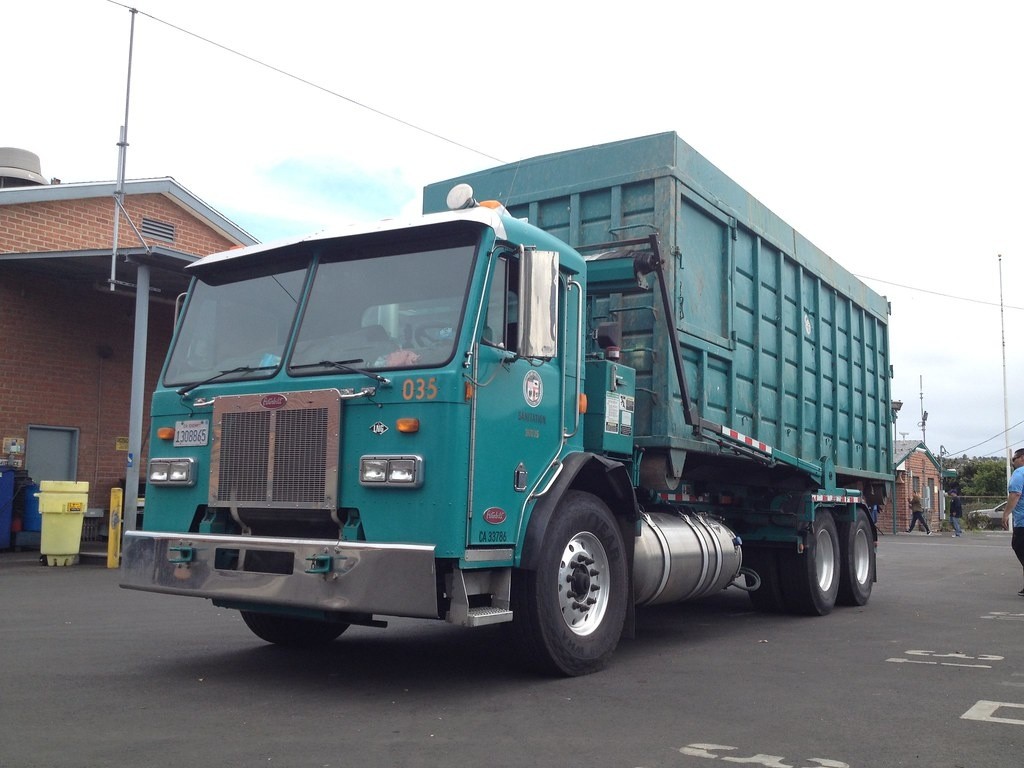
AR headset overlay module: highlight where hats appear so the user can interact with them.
[947,489,956,494]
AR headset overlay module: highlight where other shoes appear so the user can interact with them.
[927,531,932,536]
[960,531,964,535]
[952,533,958,537]
[1018,589,1023,596]
[905,531,910,533]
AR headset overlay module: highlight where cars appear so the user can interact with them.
[966,501,1008,531]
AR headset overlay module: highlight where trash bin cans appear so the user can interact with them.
[0,465,18,549]
[34,481,90,567]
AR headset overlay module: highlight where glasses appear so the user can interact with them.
[1012,455,1020,462]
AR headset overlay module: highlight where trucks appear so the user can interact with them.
[117,128,899,679]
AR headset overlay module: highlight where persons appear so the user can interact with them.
[948,489,962,537]
[905,490,932,535]
[869,504,884,524]
[1002,448,1024,596]
